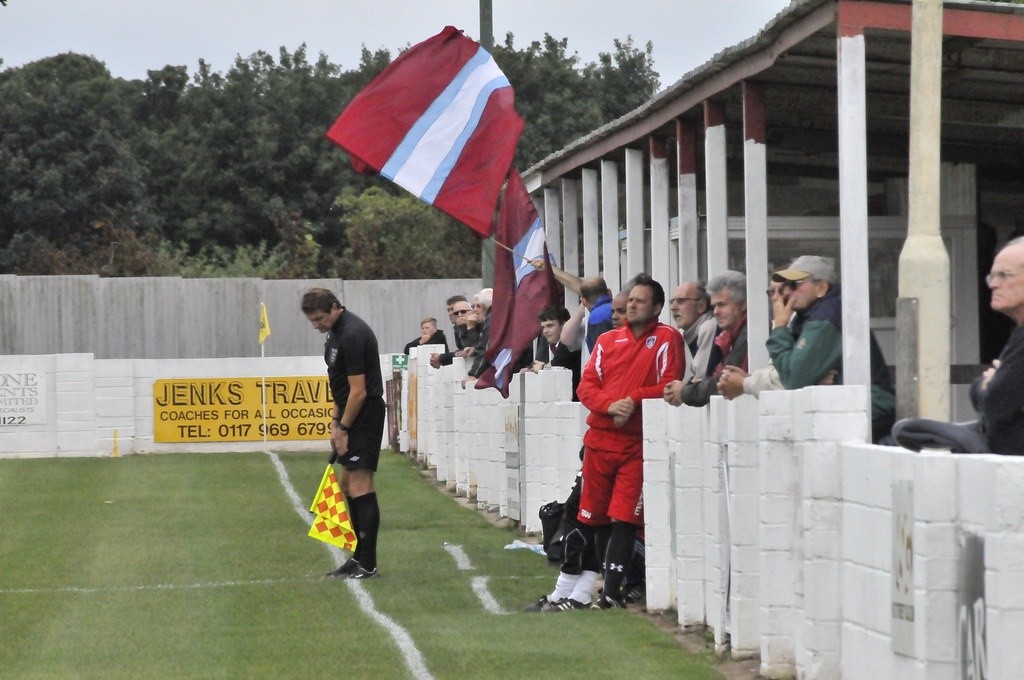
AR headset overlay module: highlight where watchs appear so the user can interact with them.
[338,423,350,432]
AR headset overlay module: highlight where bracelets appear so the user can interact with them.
[332,417,340,421]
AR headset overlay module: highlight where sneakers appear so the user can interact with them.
[592,585,645,611]
[523,593,593,612]
[326,556,379,579]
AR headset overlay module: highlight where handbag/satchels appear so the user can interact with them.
[538,501,565,554]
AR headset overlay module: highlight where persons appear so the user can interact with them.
[967,234,1024,455]
[524,277,684,615]
[404,254,898,443]
[300,287,385,579]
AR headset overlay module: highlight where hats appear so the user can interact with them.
[474,288,493,308]
[772,255,836,285]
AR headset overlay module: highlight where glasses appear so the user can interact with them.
[471,303,481,309]
[670,296,702,304]
[452,309,471,315]
[781,280,812,291]
[986,270,1021,284]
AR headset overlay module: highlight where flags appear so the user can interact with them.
[306,463,358,552]
[328,26,527,236]
[258,303,270,344]
[473,162,561,401]
